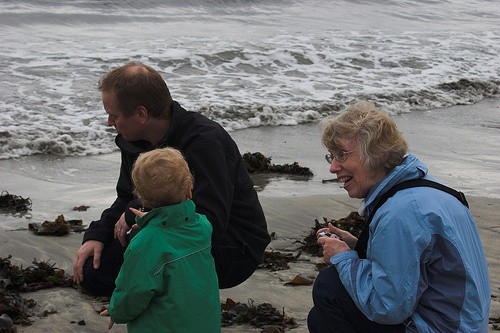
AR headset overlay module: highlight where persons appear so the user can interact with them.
[71,59,273,296]
[308,101,491,333]
[98,147,221,332]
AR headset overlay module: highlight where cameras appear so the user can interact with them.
[318,231,343,246]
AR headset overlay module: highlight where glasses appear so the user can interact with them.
[324,148,358,163]
